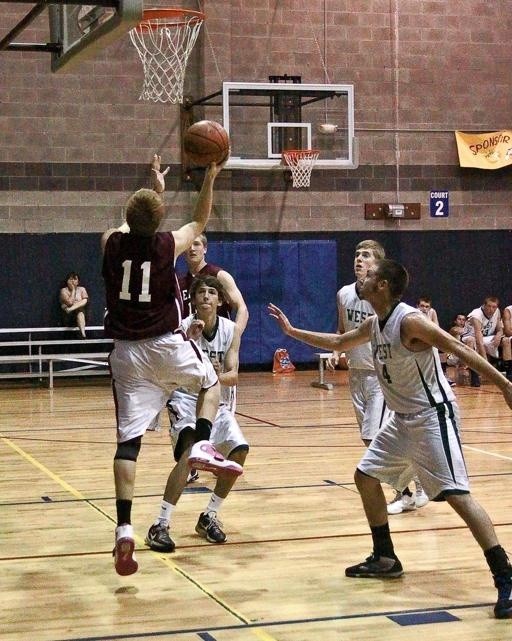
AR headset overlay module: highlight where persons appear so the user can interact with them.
[325,239,429,515]
[267,258,512,619]
[60,272,89,340]
[416,296,512,387]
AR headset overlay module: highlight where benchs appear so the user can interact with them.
[1,324,118,390]
[310,350,470,390]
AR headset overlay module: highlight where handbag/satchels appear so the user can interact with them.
[272,348,296,374]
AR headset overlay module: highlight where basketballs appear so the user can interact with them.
[184,120,228,168]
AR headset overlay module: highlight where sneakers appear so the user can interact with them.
[186,439,245,477]
[414,479,429,509]
[187,470,200,484]
[505,368,512,384]
[343,551,405,580]
[80,334,88,340]
[194,510,228,545]
[470,372,480,387]
[445,378,457,388]
[143,523,176,554]
[387,490,416,515]
[489,567,512,619]
[111,526,139,576]
